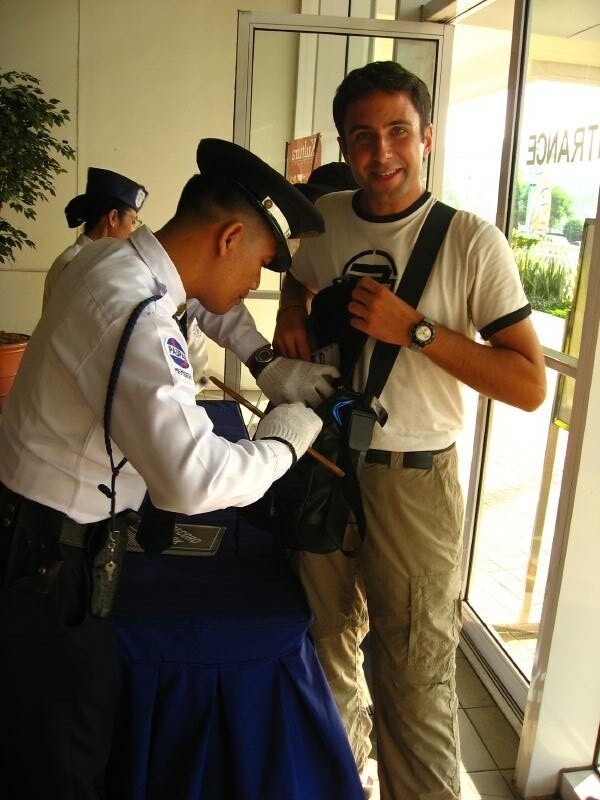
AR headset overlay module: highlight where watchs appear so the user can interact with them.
[408,316,436,352]
[249,348,278,378]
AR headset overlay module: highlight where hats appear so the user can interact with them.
[294,162,357,198]
[197,138,327,273]
[85,166,148,213]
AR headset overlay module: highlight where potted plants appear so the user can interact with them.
[0,70,75,407]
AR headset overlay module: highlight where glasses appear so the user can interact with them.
[122,213,143,229]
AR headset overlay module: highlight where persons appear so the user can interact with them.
[272,62,546,800]
[0,137,341,800]
[292,161,361,205]
[42,167,148,314]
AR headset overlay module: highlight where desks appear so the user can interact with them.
[111,399,366,800]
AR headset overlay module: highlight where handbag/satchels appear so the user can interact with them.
[250,385,370,557]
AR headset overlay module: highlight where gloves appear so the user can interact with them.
[254,402,323,461]
[255,356,336,406]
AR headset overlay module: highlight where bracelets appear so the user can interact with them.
[278,303,308,315]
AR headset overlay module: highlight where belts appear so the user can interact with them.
[1,493,89,550]
[351,442,456,470]
